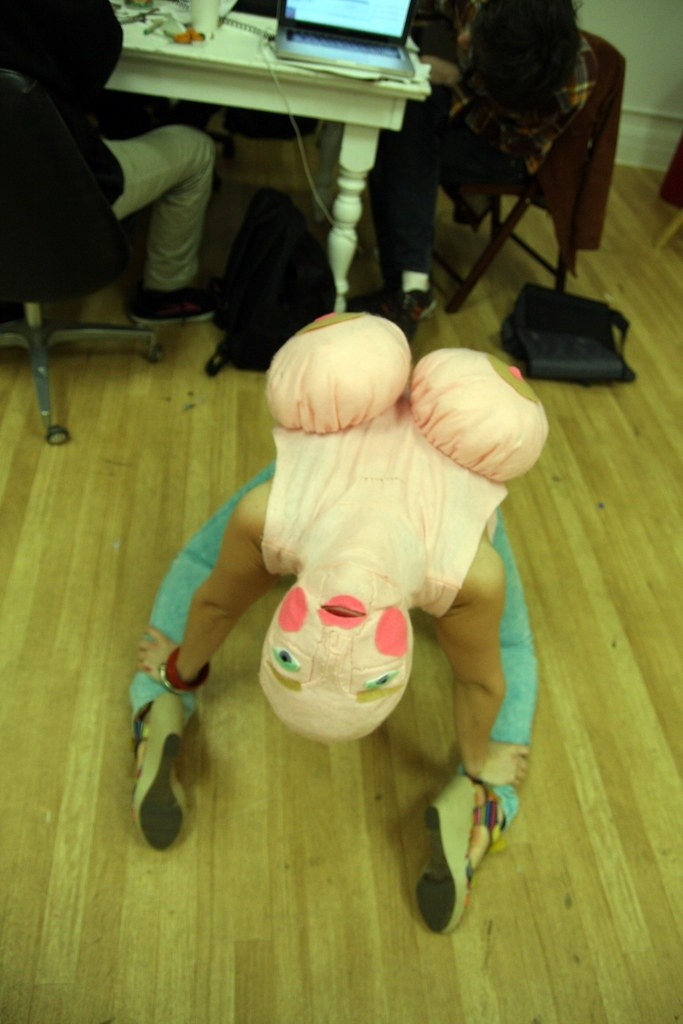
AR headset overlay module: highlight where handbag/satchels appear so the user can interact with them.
[502,281,635,384]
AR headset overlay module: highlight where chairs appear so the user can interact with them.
[0,68,161,446]
[432,0,630,314]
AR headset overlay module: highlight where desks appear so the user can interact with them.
[108,0,431,313]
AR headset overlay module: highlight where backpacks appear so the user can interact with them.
[206,189,336,376]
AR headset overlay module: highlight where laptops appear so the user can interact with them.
[275,0,416,78]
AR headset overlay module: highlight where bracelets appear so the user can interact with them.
[159,648,209,695]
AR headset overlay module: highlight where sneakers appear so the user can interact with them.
[127,279,217,327]
[378,287,437,344]
[347,285,391,315]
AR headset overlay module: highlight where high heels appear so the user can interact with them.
[417,773,475,937]
[136,694,191,850]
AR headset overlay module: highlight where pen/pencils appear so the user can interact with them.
[144,19,163,34]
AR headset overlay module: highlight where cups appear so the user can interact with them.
[191,0,219,41]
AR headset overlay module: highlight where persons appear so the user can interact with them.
[365,0,594,320]
[0,0,217,326]
[126,312,549,933]
